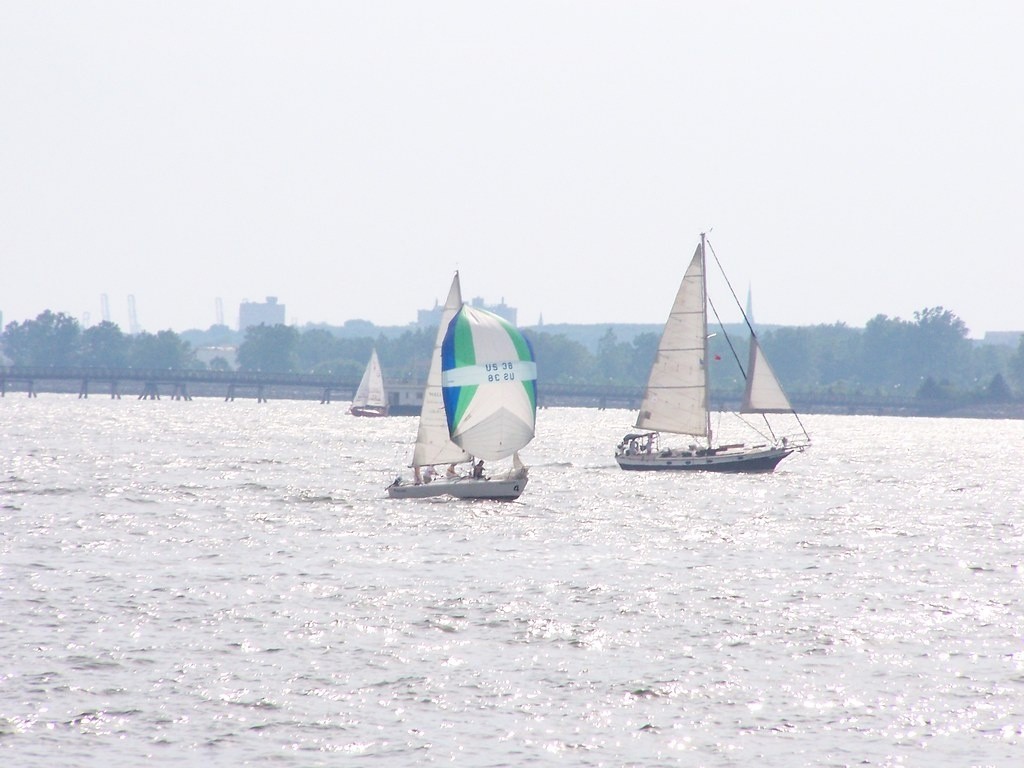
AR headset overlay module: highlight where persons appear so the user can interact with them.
[629,438,638,454]
[446,463,459,480]
[646,433,660,455]
[474,460,485,477]
[423,465,438,484]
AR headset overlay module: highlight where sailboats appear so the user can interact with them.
[347,347,392,417]
[611,232,812,471]
[380,270,540,501]
